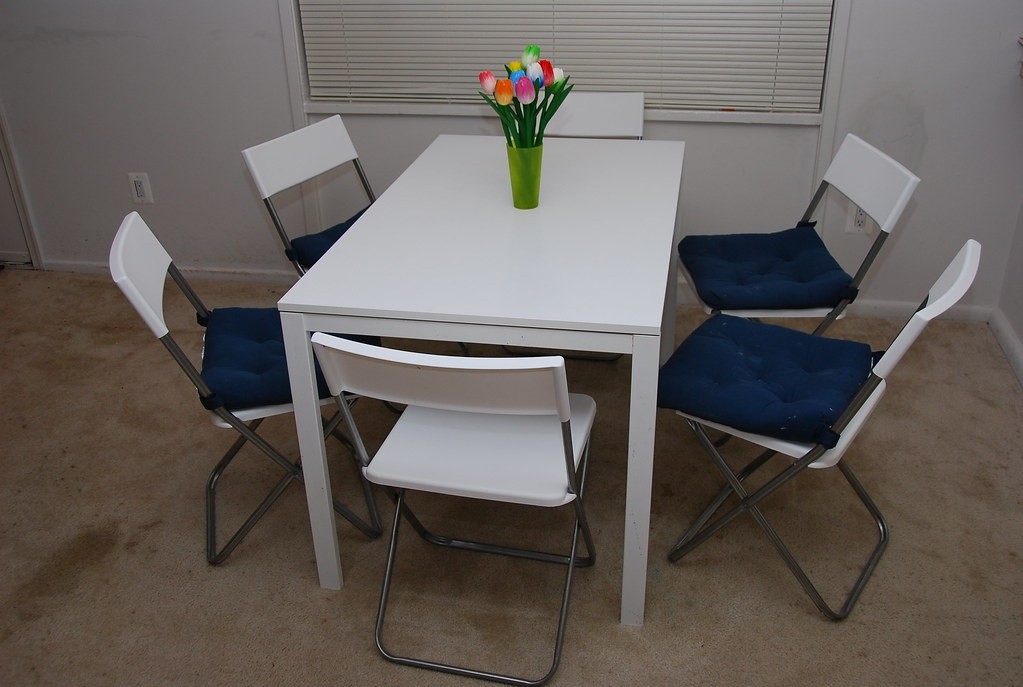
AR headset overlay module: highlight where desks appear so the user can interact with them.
[277,132,693,627]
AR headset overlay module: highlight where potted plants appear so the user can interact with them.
[506,141,544,214]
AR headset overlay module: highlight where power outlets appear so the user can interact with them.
[129,172,155,207]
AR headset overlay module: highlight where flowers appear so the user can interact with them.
[477,43,575,149]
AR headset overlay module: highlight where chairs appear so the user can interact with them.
[523,85,648,141]
[106,210,393,567]
[675,133,920,324]
[243,114,386,281]
[657,237,987,623]
[306,331,601,683]
